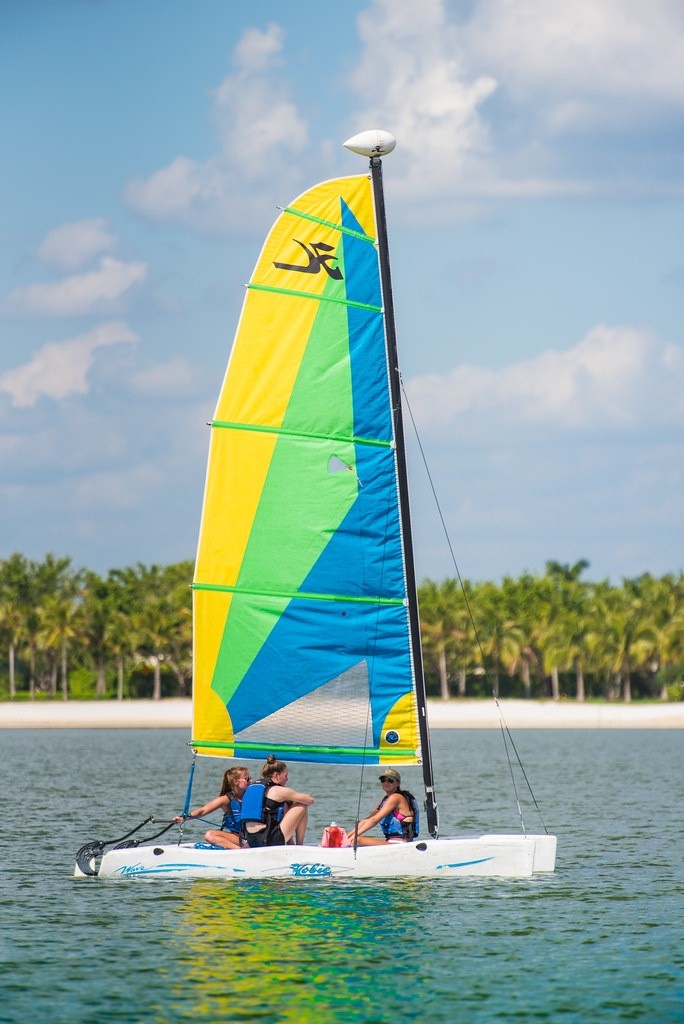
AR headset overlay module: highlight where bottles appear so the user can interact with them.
[329,822,340,847]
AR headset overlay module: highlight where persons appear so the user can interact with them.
[348,769,414,847]
[173,766,295,850]
[241,754,315,848]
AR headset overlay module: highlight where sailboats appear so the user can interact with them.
[73,128,558,885]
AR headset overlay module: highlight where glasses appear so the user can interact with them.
[381,778,395,783]
[238,777,251,782]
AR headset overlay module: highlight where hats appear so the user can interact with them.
[378,769,401,782]
